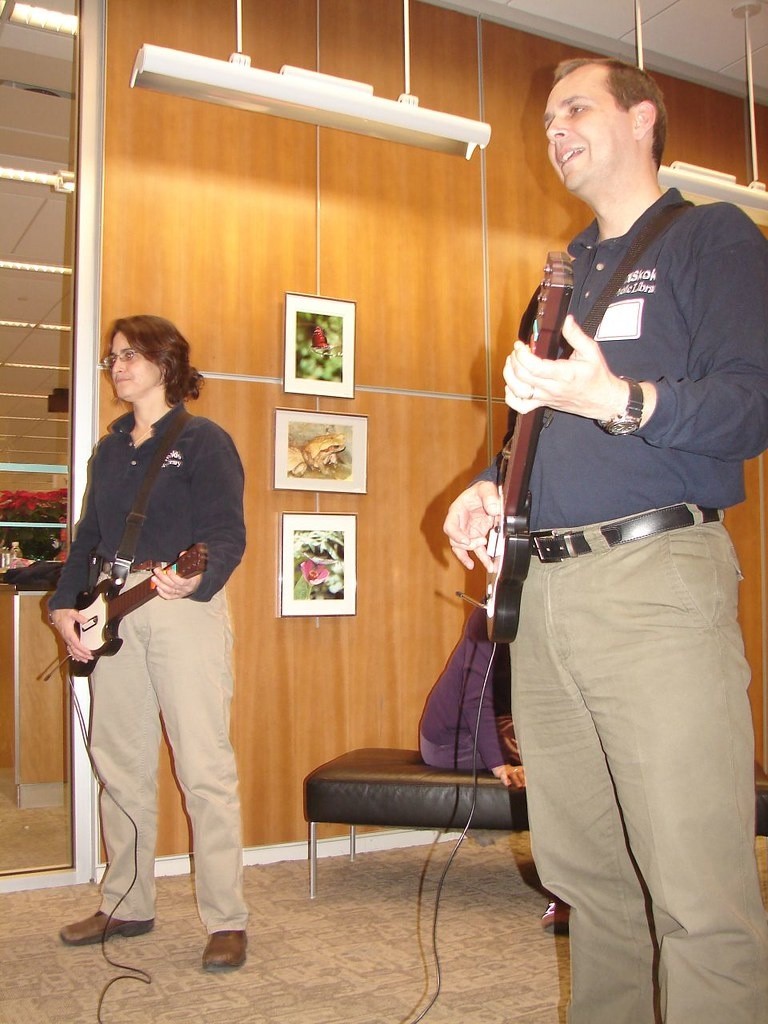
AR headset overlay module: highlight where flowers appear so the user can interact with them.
[0,487,68,561]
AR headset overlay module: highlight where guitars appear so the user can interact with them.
[68,541,209,677]
[484,251,575,643]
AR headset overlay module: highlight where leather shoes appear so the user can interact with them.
[59,910,154,944]
[201,930,246,969]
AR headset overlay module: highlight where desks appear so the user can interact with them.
[0,583,68,809]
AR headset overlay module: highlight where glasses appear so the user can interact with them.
[104,348,139,368]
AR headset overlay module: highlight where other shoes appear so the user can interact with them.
[542,899,571,935]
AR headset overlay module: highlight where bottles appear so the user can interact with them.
[10,542,22,564]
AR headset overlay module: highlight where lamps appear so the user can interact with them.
[621,0,768,225]
[128,1,492,163]
[0,0,79,37]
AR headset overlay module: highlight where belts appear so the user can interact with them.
[100,559,173,577]
[530,502,719,564]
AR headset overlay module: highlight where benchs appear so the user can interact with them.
[302,747,768,901]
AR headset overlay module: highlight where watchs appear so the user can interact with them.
[598,376,644,438]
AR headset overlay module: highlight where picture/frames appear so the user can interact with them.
[282,292,357,400]
[272,407,369,496]
[276,510,358,618]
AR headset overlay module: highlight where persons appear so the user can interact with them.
[445,56,768,1024]
[45,317,247,975]
[418,599,526,786]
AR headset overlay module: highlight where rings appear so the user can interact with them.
[68,643,73,647]
[527,385,535,401]
[514,769,518,773]
[174,588,178,595]
